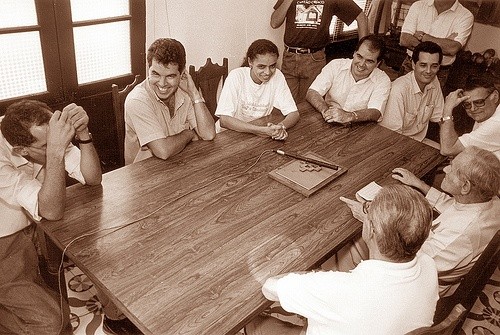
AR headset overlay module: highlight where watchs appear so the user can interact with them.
[438,115,454,125]
[74,132,94,144]
[352,111,358,123]
[192,98,205,105]
[417,32,426,42]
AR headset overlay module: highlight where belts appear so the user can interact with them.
[284,44,325,54]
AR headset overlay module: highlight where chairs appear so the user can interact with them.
[407,300,467,335]
[440,230,500,313]
[111,74,144,163]
[188,56,228,123]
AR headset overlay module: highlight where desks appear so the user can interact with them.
[21,98,449,335]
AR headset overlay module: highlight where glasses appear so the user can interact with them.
[461,88,500,109]
[362,200,375,229]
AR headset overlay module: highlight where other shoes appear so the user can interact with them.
[260,301,306,327]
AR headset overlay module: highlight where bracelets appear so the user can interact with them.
[278,122,287,129]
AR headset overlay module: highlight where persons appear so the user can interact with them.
[306,34,391,126]
[440,73,500,160]
[380,41,444,145]
[270,0,369,105]
[399,0,474,92]
[213,39,300,140]
[124,37,216,166]
[316,145,500,296]
[259,184,440,335]
[0,98,102,335]
